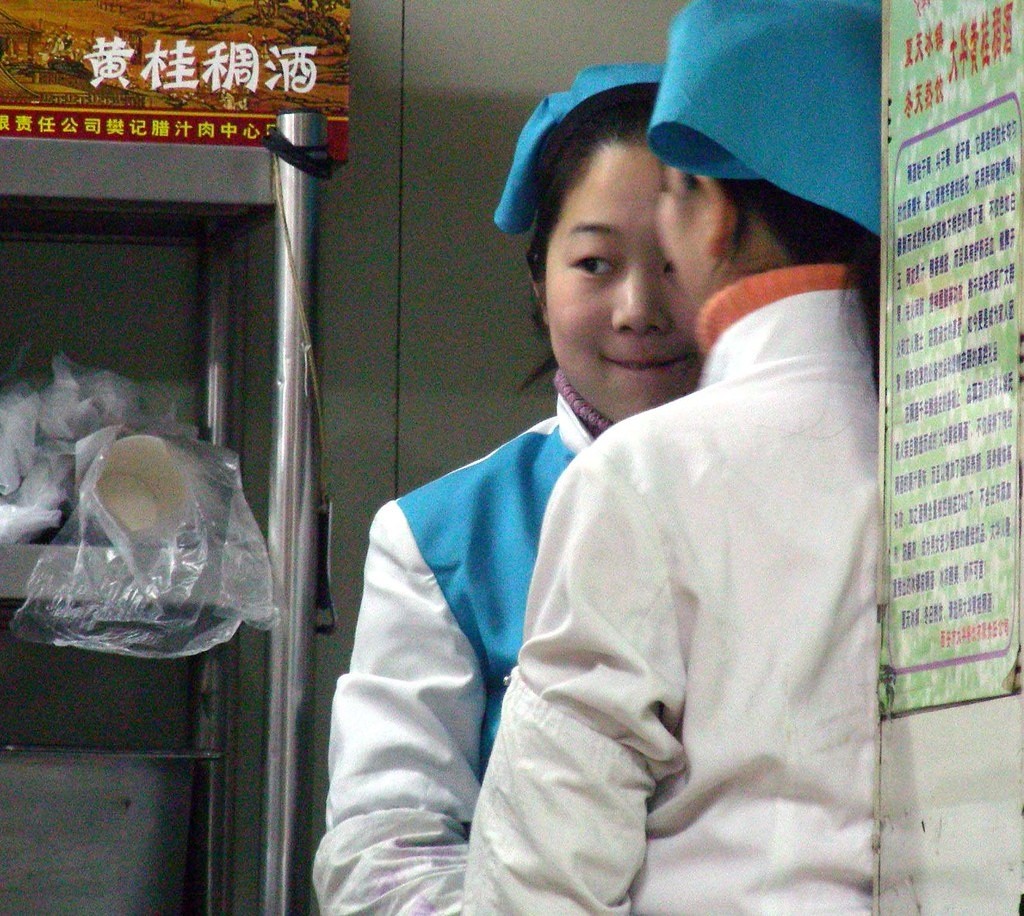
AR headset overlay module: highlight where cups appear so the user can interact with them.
[97,435,187,531]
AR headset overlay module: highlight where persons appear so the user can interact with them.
[312,62,704,915]
[460,3,883,916]
